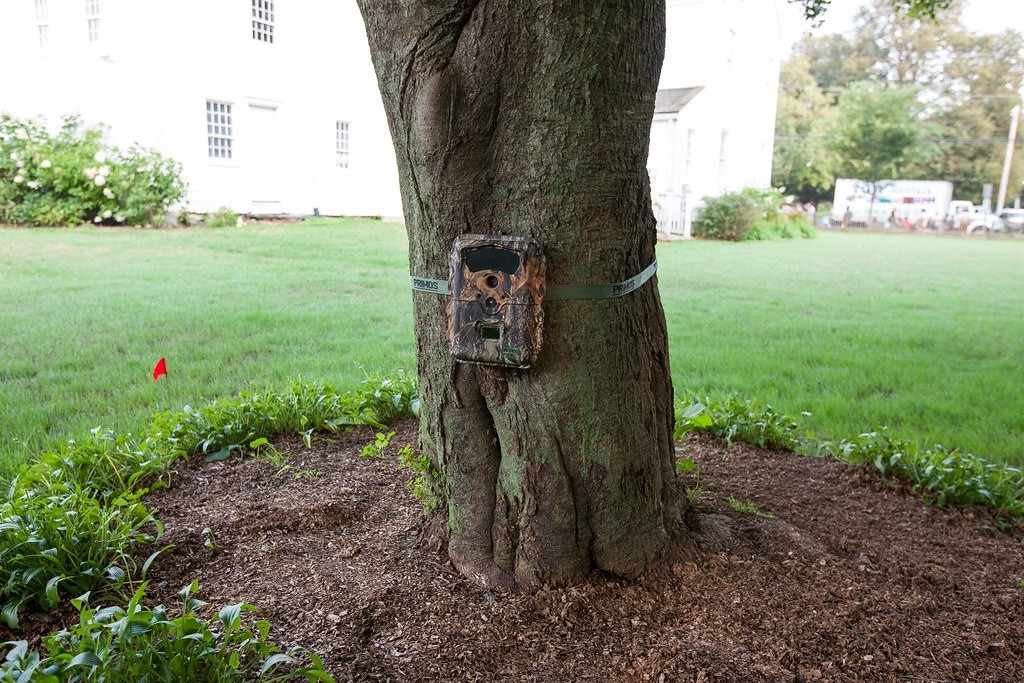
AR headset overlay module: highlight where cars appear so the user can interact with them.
[996,208,1024,231]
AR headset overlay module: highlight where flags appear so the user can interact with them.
[153,358,167,380]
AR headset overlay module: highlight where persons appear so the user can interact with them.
[844,206,852,226]
[921,209,927,226]
[807,202,816,223]
[889,207,896,221]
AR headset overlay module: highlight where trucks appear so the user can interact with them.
[832,177,975,229]
